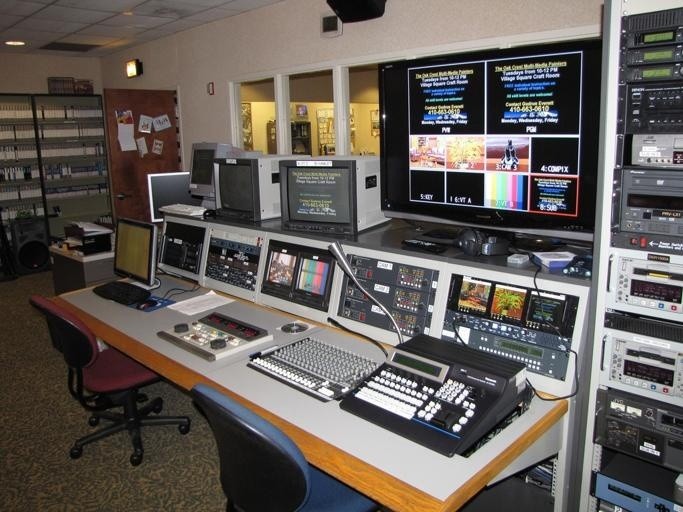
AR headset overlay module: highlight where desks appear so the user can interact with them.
[47,242,115,296]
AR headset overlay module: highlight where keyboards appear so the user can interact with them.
[93,281,151,305]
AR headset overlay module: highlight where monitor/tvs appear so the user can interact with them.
[189,143,263,197]
[279,155,392,236]
[113,217,158,286]
[214,155,310,223]
[378,37,602,244]
[146,172,193,223]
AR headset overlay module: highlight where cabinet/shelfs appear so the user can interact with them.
[267,120,312,156]
[0,92,114,242]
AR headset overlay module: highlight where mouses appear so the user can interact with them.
[136,300,157,310]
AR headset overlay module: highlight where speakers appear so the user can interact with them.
[326,1,386,24]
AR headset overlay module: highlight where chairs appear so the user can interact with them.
[189,382,380,512]
[27,292,192,468]
